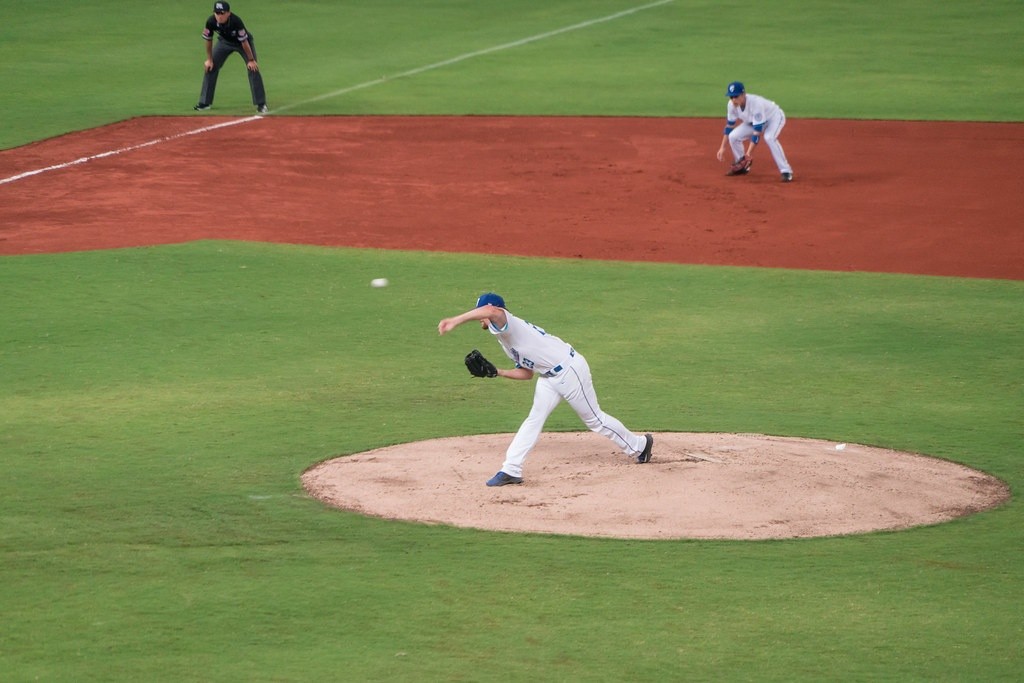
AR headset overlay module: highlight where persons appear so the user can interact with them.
[716,81,793,182]
[437,293,654,486]
[193,0,268,113]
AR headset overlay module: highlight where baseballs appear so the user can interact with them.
[370,278,388,287]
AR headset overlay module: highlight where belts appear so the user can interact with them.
[546,349,574,377]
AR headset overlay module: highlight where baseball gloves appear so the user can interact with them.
[731,155,752,175]
[465,349,498,378]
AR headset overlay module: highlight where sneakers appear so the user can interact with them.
[783,172,792,181]
[727,167,750,176]
[257,103,268,114]
[637,434,653,464]
[487,471,522,487]
[193,102,211,110]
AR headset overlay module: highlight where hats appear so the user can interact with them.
[213,1,230,12]
[726,82,744,96]
[475,293,505,308]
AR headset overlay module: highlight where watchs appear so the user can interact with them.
[248,59,255,61]
